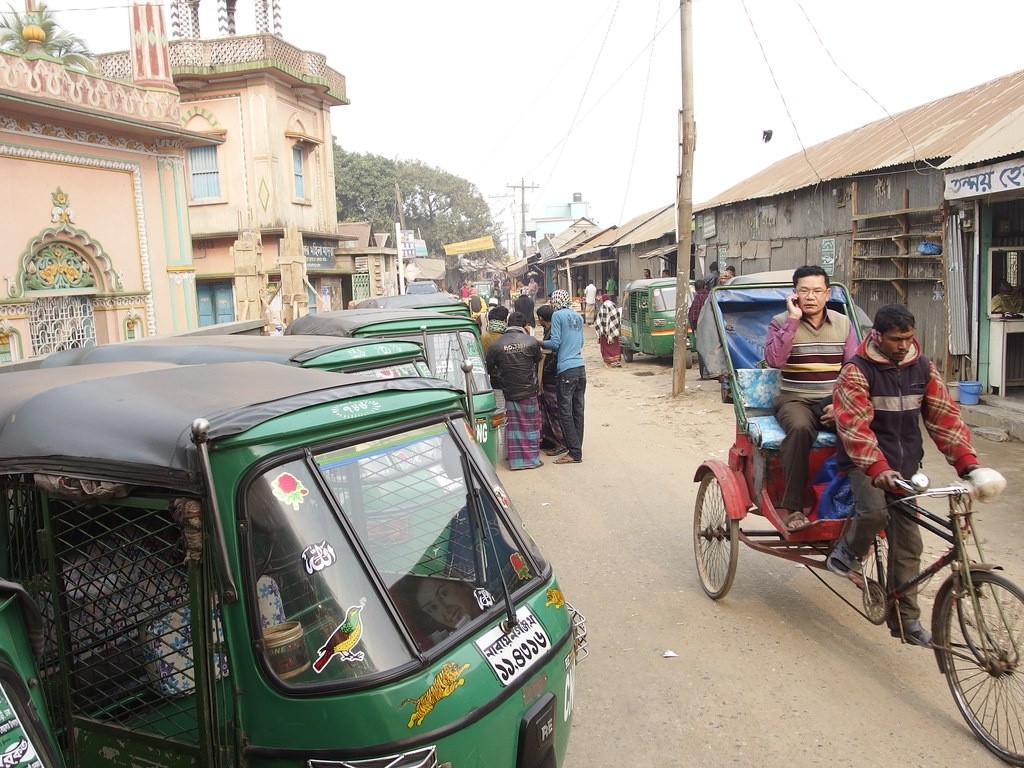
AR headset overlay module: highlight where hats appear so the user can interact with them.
[489,297,499,305]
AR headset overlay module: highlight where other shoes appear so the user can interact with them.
[540,441,552,450]
[547,447,568,457]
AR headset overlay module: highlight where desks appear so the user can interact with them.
[988,319,1024,397]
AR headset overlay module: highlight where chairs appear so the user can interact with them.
[138,574,285,698]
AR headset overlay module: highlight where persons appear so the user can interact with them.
[460,283,477,298]
[595,294,621,367]
[494,283,511,306]
[519,277,538,307]
[689,262,736,380]
[992,279,1024,313]
[644,269,651,279]
[662,270,670,278]
[404,575,481,631]
[764,265,858,531]
[828,303,1007,648]
[480,289,587,469]
[585,279,603,325]
[605,275,617,300]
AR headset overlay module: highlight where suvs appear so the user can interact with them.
[406,280,439,297]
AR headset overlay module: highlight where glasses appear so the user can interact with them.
[794,287,828,299]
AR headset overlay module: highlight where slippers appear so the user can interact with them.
[553,455,580,463]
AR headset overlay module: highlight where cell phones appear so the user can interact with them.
[793,290,797,306]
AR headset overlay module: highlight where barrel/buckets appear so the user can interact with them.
[946,381,983,405]
[262,621,311,681]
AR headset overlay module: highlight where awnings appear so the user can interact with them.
[505,254,545,273]
[639,243,694,265]
[558,258,618,281]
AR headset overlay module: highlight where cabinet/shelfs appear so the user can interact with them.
[849,202,951,305]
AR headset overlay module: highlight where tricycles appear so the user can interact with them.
[694,280,1024,768]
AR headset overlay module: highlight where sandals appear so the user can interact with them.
[786,512,809,529]
[891,619,933,649]
[827,540,859,578]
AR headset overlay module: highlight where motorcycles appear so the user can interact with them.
[284,306,509,473]
[619,277,698,369]
[354,291,473,319]
[472,281,494,309]
[38,334,468,576]
[0,361,591,768]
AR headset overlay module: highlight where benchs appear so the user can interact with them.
[734,368,838,511]
[16,503,285,680]
